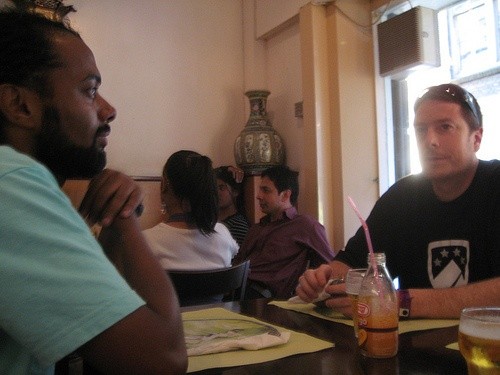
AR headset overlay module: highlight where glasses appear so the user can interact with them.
[416,85,479,118]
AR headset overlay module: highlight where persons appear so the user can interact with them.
[298,83,500,320]
[214,166,249,248]
[142,151,239,313]
[0,0,188,375]
[233,166,333,312]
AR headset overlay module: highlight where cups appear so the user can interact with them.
[459,306,500,375]
[347,268,368,337]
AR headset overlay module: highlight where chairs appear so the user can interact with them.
[168,256,251,307]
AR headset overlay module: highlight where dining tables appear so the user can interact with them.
[181,295,470,375]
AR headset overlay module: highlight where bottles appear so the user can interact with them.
[357,252,399,359]
[233,89,285,176]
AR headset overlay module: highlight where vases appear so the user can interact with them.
[236,88,287,169]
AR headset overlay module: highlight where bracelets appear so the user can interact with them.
[399,290,410,319]
